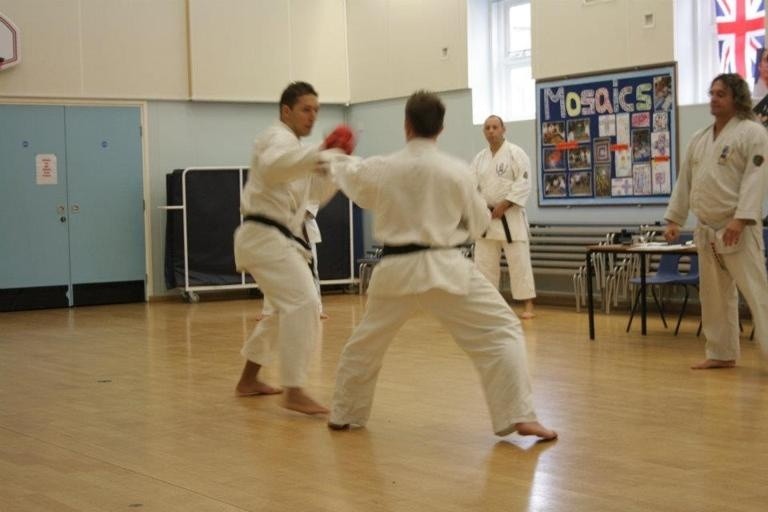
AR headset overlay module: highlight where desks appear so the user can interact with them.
[583,243,700,340]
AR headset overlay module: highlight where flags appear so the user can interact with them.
[714,0,765,88]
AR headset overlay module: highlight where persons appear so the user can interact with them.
[472,115,537,320]
[754,49,768,93]
[303,91,558,440]
[664,73,768,374]
[235,81,354,414]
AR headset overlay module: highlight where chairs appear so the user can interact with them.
[623,233,714,336]
